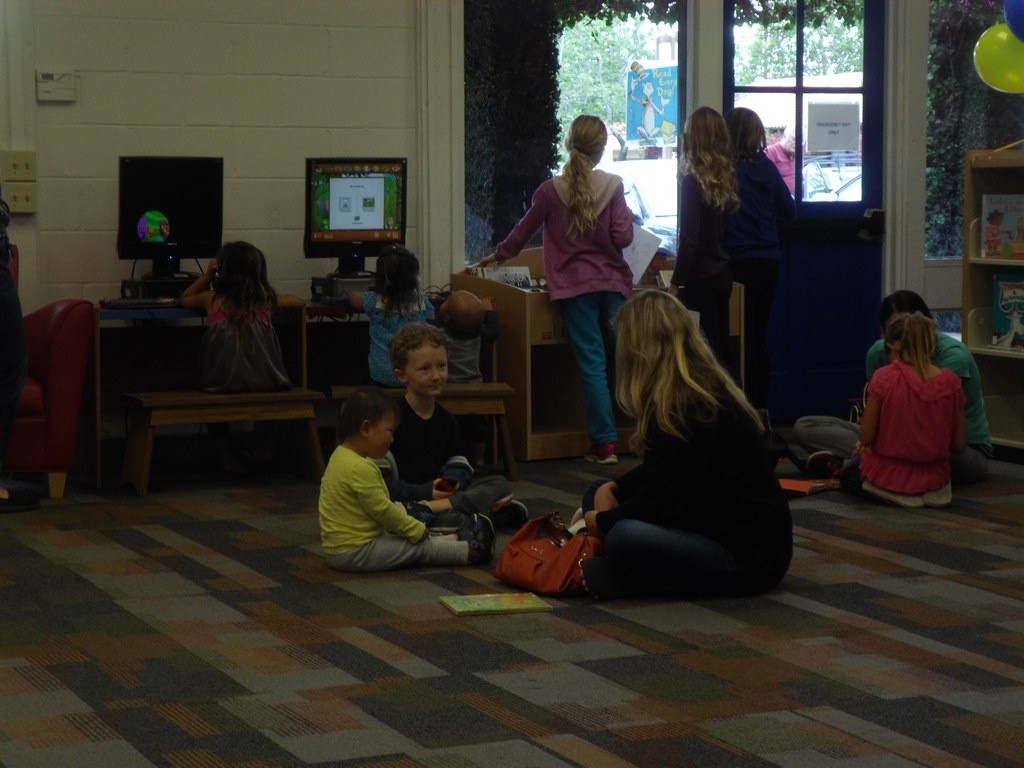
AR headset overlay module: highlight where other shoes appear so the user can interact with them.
[454,514,477,540]
[397,499,437,528]
[925,476,952,507]
[491,500,528,527]
[860,481,926,509]
[469,512,494,562]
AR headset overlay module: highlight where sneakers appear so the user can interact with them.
[584,440,618,464]
[807,451,844,478]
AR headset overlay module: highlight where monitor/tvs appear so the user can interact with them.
[304,157,407,279]
[117,156,224,281]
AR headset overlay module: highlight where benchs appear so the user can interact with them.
[122,388,327,497]
[329,381,520,481]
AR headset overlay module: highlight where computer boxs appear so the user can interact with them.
[311,276,381,305]
[121,279,201,300]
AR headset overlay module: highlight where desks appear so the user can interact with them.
[95,294,306,490]
[305,299,500,475]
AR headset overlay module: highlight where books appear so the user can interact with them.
[439,592,554,616]
[980,194,1024,350]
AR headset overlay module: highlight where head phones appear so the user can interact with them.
[214,244,232,287]
[375,247,418,277]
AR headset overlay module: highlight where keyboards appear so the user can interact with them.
[99,298,182,308]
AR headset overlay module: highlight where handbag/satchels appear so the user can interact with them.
[495,511,600,597]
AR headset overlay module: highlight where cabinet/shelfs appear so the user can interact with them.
[449,246,745,463]
[962,149,1024,451]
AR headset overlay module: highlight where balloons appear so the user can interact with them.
[973,0,1024,94]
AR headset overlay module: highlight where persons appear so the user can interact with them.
[571,289,793,603]
[791,290,993,509]
[478,115,637,465]
[0,198,41,514]
[764,122,797,198]
[180,243,293,470]
[667,107,796,390]
[318,247,530,573]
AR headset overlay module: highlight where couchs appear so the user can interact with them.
[9,299,95,499]
